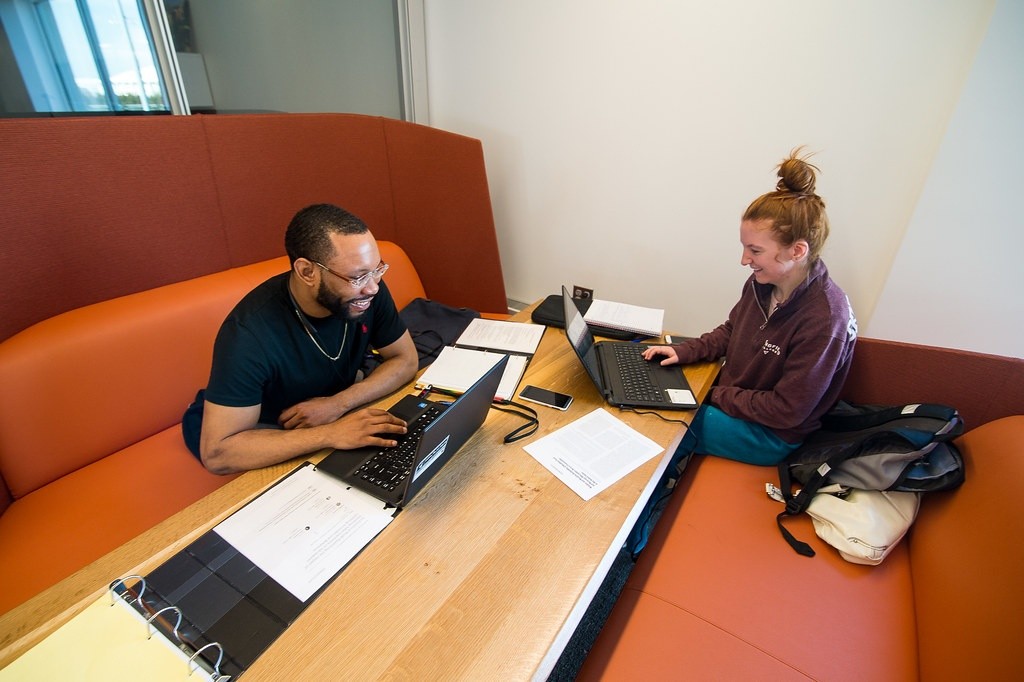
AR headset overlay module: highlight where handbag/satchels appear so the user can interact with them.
[361,298,481,379]
[764,483,922,565]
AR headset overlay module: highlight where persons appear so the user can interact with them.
[182,204,421,475]
[621,158,859,555]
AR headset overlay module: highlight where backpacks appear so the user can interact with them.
[778,403,966,558]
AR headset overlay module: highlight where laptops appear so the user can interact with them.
[561,284,699,411]
[314,354,512,509]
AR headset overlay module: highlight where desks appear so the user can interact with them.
[0,298,727,682]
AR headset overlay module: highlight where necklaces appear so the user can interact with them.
[287,282,348,361]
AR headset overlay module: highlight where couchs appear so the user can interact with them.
[0,241,514,616]
[578,413,1024,682]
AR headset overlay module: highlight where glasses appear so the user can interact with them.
[310,259,389,287]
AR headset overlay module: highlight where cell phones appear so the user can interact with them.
[518,384,573,411]
